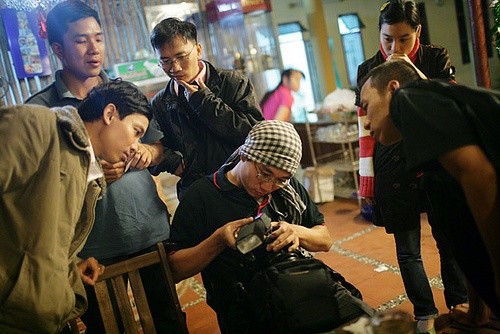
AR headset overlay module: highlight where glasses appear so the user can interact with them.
[253,161,291,189]
[157,42,197,69]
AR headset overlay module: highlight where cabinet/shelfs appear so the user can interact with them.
[303,107,364,209]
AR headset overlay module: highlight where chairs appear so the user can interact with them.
[69,241,189,334]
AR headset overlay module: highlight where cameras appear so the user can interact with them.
[235,214,302,264]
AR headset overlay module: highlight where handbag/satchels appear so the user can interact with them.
[248,258,364,333]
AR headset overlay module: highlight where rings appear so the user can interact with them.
[98,267,100,271]
[136,151,141,156]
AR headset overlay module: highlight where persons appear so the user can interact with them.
[150,17,265,200]
[357,60,500,334]
[260,69,301,123]
[23,0,189,334]
[0,77,154,334]
[164,120,363,334]
[355,0,469,334]
